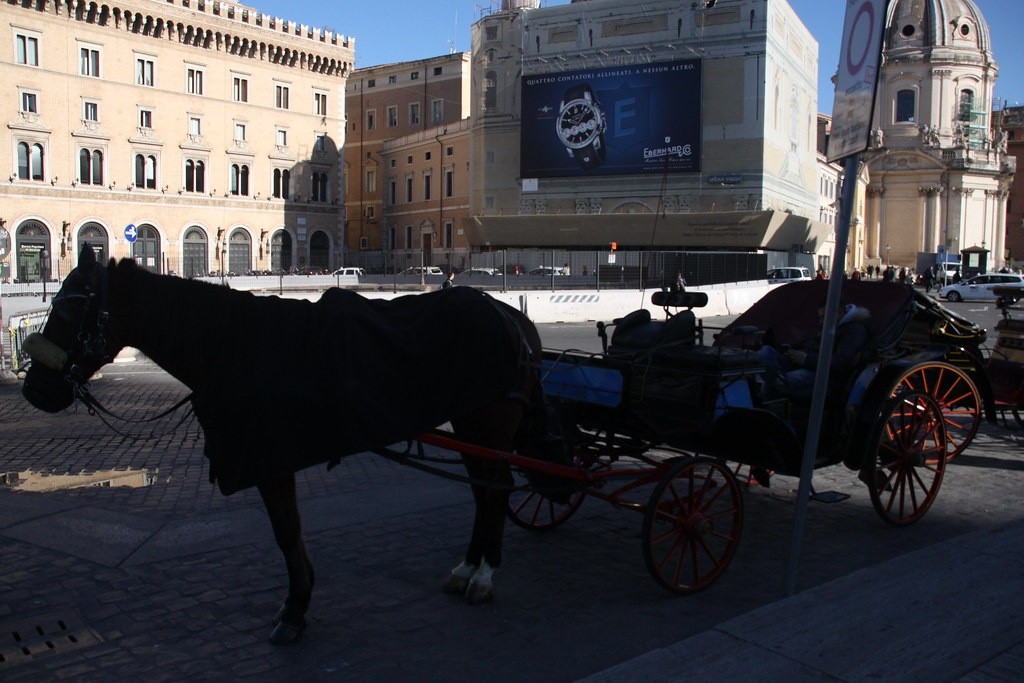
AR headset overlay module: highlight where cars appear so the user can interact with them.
[939,273,1024,303]
[293,266,330,275]
[459,267,502,275]
[329,267,366,275]
[936,263,962,283]
[397,267,443,275]
[528,265,563,275]
[767,267,812,284]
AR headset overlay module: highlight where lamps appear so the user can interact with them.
[109,180,116,190]
[254,192,260,200]
[10,173,17,183]
[162,185,169,194]
[225,190,232,198]
[178,187,185,195]
[294,193,301,201]
[261,229,270,254]
[218,227,226,253]
[268,193,275,200]
[127,182,135,191]
[432,232,437,237]
[63,220,73,250]
[72,178,79,188]
[51,176,58,185]
[209,189,216,196]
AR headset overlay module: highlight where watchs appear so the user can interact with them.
[556,81,607,170]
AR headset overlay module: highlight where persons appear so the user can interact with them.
[742,297,871,486]
[843,271,847,279]
[865,264,880,279]
[924,266,935,293]
[999,128,1008,149]
[871,126,883,146]
[559,263,570,277]
[442,272,454,289]
[920,123,941,145]
[815,264,827,280]
[883,266,921,285]
[954,123,966,145]
[852,268,861,281]
[999,267,1008,273]
[953,270,963,284]
[674,271,686,287]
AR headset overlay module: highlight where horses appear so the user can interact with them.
[20,239,546,645]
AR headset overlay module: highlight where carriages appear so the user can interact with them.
[20,240,990,647]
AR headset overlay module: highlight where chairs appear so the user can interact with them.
[608,309,719,366]
